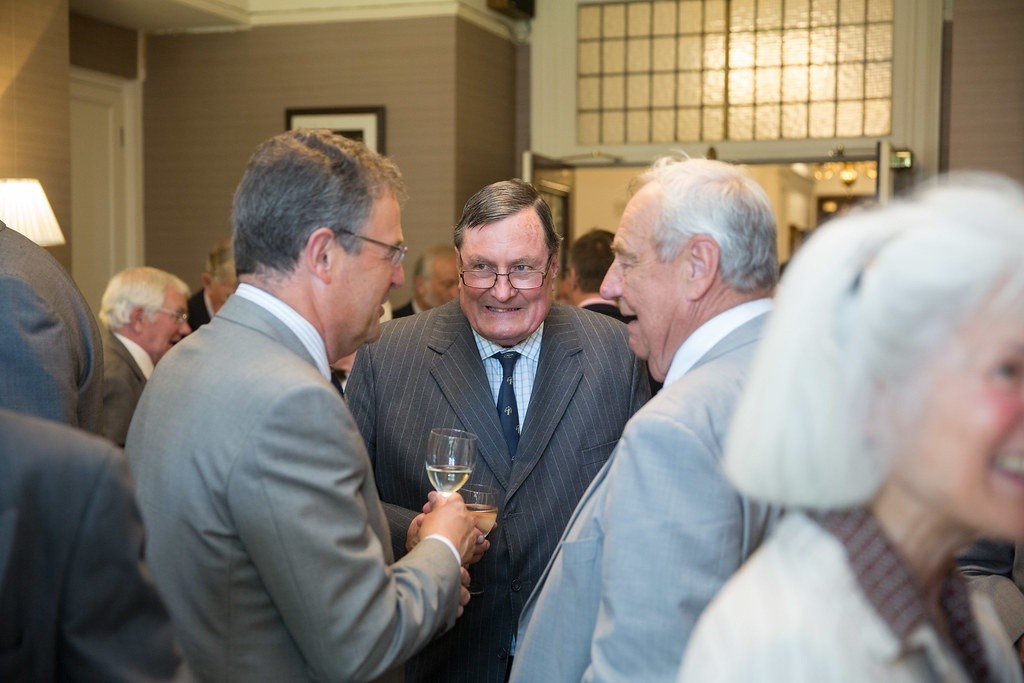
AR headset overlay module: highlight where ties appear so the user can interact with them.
[489,351,521,464]
[330,371,344,401]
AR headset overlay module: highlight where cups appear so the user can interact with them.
[459,484,499,539]
[425,428,478,497]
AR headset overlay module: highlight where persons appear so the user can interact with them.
[0,408,185,683]
[704,167,1024,683]
[0,219,105,439]
[345,176,653,683]
[99,230,793,448]
[505,150,787,683]
[124,128,478,683]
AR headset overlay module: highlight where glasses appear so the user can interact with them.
[303,227,407,267]
[159,308,190,324]
[459,253,554,290]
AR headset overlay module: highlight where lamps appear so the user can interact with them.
[0,178,67,249]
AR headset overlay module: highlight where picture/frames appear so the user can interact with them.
[285,106,383,158]
